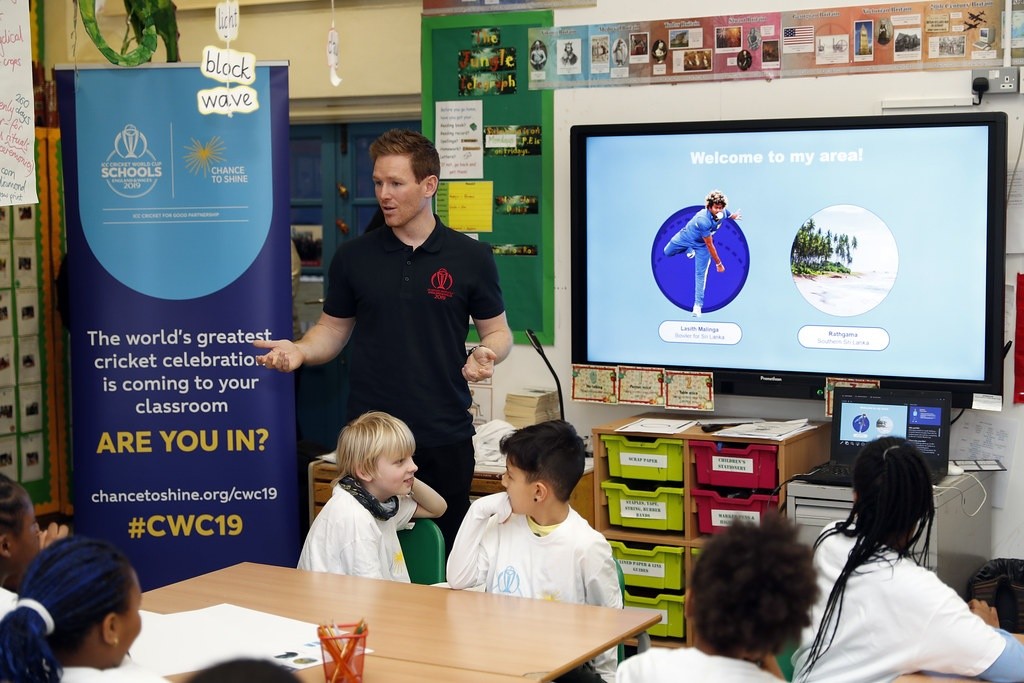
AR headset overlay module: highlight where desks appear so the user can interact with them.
[309,459,595,526]
[892,633,1024,683]
[138,561,662,683]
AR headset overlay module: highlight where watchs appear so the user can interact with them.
[467,344,486,358]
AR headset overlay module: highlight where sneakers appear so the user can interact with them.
[692,305,701,317]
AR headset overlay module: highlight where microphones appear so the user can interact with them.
[525,327,565,422]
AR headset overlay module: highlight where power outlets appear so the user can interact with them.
[972,68,1018,93]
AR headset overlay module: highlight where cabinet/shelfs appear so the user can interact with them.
[592,411,831,646]
[787,470,996,596]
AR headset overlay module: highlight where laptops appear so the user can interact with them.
[800,387,953,488]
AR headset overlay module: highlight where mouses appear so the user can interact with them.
[948,464,964,476]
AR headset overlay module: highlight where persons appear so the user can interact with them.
[295,411,448,585]
[0,473,68,620]
[253,129,513,583]
[663,191,743,318]
[0,535,142,683]
[788,436,1024,683]
[428,420,623,683]
[614,511,822,683]
[184,656,302,683]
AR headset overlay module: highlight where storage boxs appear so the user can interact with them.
[607,539,685,590]
[601,477,684,532]
[624,590,686,639]
[690,487,779,535]
[600,435,683,482]
[688,440,778,489]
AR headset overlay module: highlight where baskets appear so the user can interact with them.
[317,623,368,683]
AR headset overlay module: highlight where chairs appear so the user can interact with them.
[396,517,445,582]
[615,561,626,665]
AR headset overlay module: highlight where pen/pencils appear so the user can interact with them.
[318,617,366,683]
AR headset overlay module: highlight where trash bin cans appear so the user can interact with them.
[970,558,1024,634]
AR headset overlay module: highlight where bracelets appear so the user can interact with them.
[716,263,721,266]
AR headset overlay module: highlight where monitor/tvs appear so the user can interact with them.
[571,111,1009,421]
